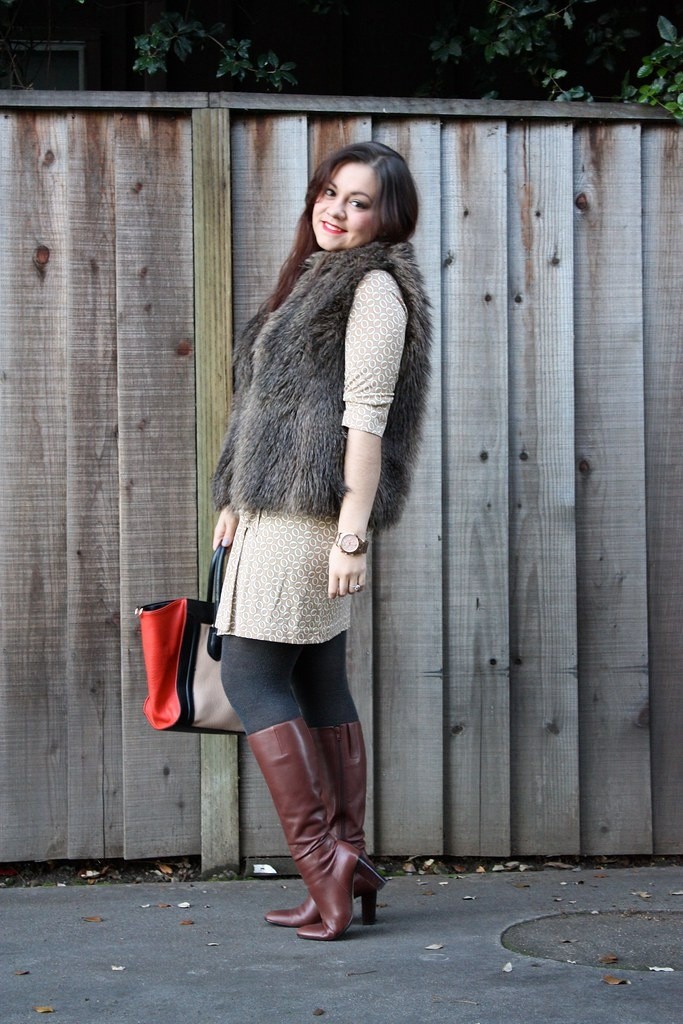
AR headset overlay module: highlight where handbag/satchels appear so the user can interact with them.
[133,538,248,735]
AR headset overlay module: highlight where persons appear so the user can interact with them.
[212,141,429,940]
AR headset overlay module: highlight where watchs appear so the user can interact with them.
[334,531,369,555]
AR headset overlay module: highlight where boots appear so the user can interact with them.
[264,721,379,928]
[247,716,388,942]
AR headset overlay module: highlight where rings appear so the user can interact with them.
[349,585,360,591]
